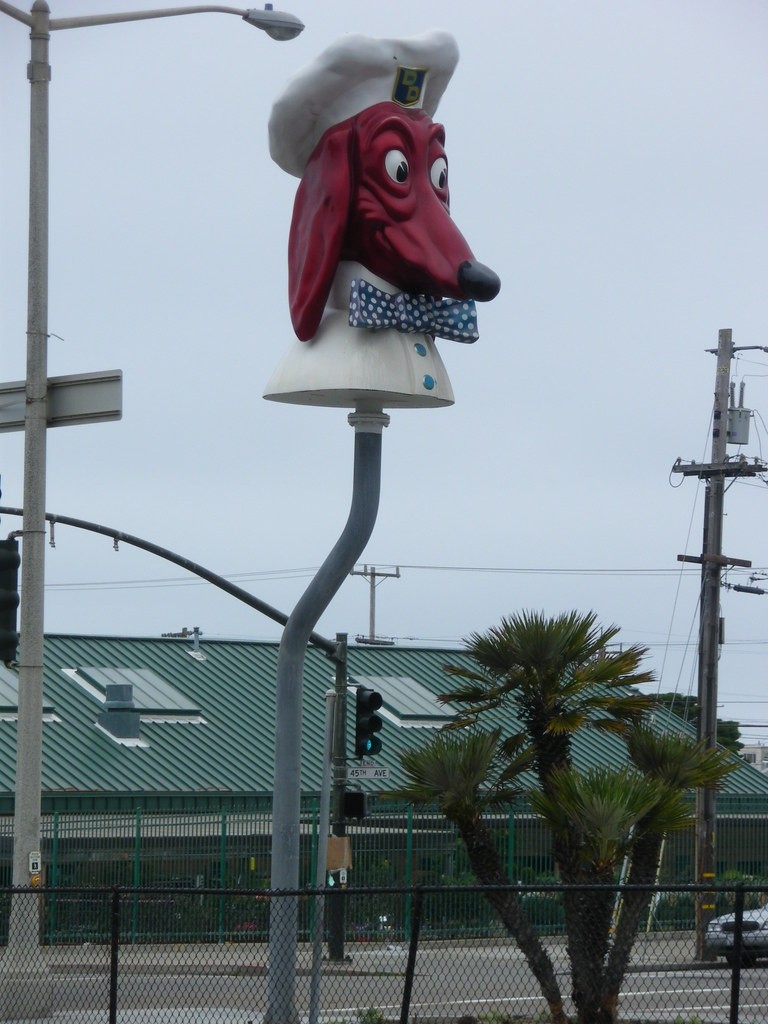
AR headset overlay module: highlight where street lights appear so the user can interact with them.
[0,0,305,1021]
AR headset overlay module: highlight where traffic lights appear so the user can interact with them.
[355,686,384,758]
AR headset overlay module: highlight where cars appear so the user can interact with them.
[703,902,768,969]
